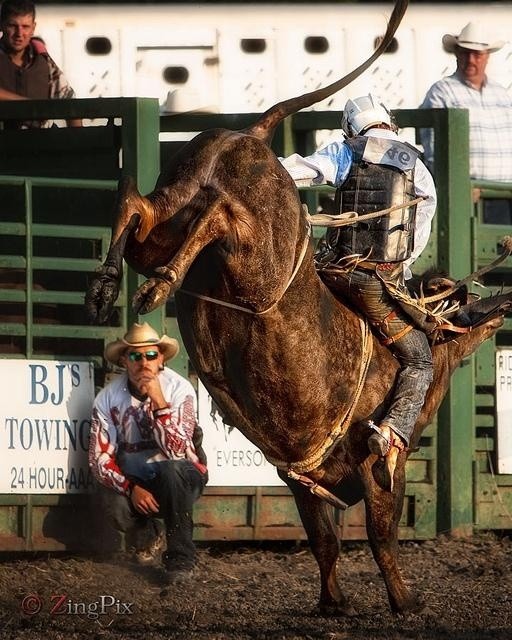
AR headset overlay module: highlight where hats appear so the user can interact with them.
[160,90,220,116]
[105,321,179,369]
[442,21,505,53]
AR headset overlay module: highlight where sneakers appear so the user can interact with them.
[126,531,165,566]
[368,425,405,492]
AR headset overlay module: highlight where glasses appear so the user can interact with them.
[129,351,158,361]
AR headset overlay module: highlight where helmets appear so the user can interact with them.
[341,93,392,137]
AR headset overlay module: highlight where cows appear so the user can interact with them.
[82,0,505,618]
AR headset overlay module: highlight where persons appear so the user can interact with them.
[87,322,209,601]
[0,1,83,130]
[415,20,512,286]
[276,93,434,492]
